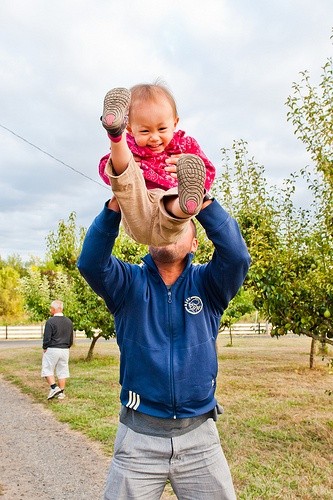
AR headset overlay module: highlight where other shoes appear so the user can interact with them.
[100,88,131,134]
[177,154,206,215]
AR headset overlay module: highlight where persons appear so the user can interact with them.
[75,187,253,500]
[98,83,217,247]
[42,300,73,399]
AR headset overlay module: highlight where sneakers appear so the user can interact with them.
[47,386,61,400]
[58,393,65,400]
